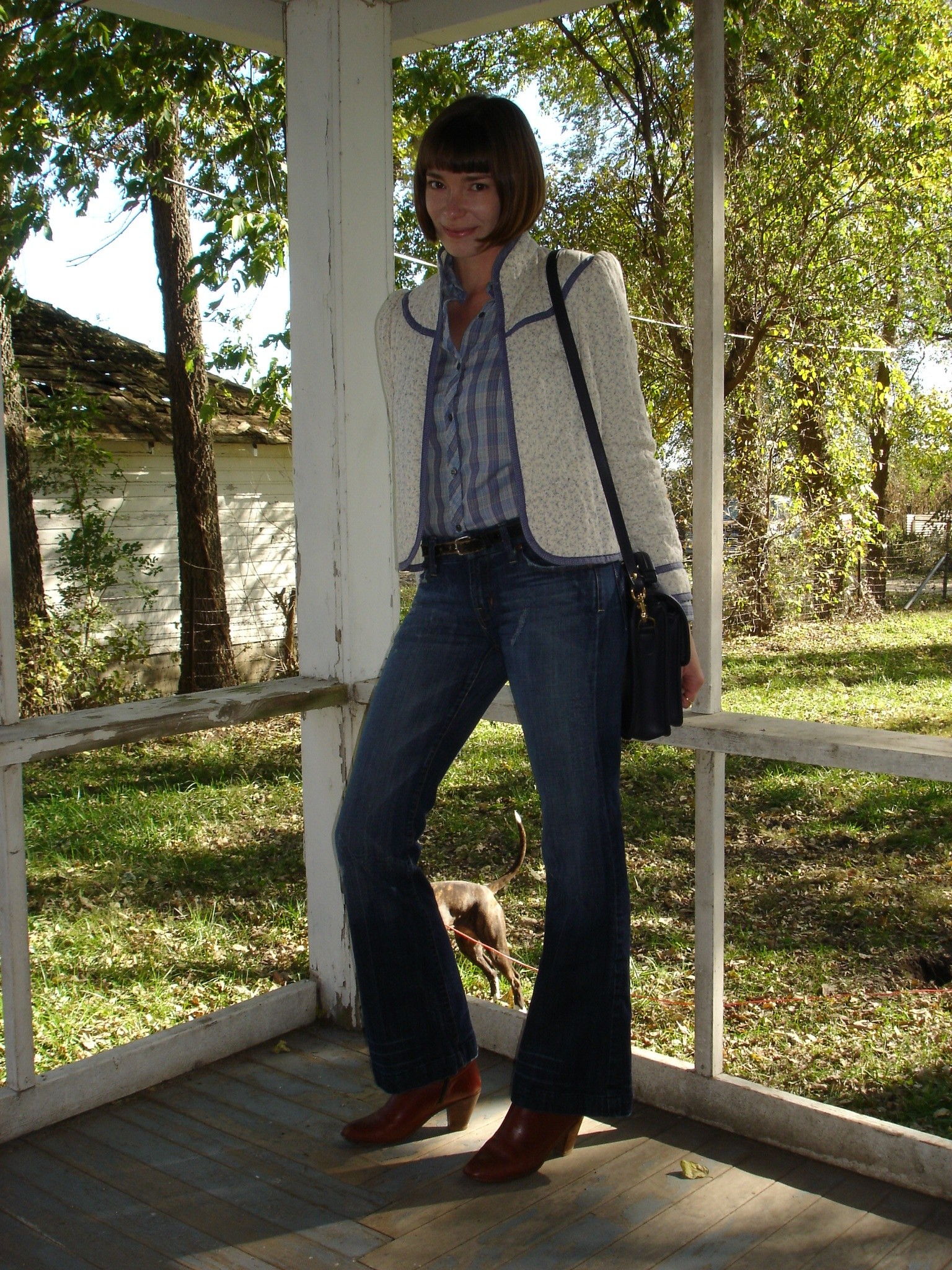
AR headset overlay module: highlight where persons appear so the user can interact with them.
[332,92,704,1181]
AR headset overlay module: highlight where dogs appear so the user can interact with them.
[432,810,526,1012]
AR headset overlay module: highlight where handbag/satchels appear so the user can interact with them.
[617,580,690,738]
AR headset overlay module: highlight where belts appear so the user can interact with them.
[430,531,490,559]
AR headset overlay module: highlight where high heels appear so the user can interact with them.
[341,1058,482,1146]
[463,1100,583,1183]
